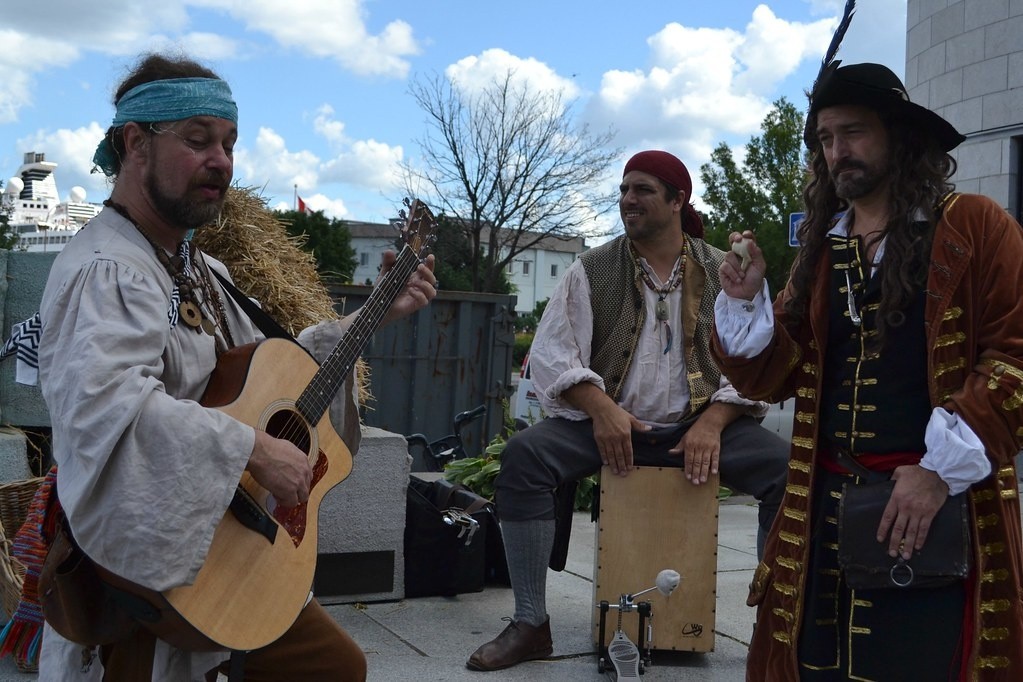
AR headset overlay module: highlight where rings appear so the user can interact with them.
[432,280,440,290]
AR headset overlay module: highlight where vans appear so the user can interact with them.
[515,349,545,428]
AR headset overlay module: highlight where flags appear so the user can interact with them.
[297,196,315,217]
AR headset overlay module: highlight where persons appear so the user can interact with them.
[464,149,795,668]
[712,58,1022,682]
[0,50,441,682]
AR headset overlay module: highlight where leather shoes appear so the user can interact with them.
[466,614,553,670]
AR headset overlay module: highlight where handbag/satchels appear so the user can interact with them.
[836,478,971,591]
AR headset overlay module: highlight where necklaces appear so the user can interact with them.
[629,230,688,355]
[102,200,236,349]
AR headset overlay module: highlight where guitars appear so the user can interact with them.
[93,197,442,655]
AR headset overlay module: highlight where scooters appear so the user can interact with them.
[405,405,488,473]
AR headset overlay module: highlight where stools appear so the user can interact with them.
[591,462,723,670]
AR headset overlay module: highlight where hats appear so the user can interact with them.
[624,150,693,206]
[812,62,966,151]
